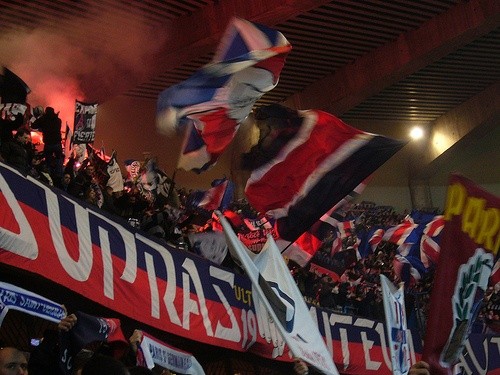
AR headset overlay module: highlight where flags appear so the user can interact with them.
[156,14,295,177]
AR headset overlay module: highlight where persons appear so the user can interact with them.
[1,97,500,375]
